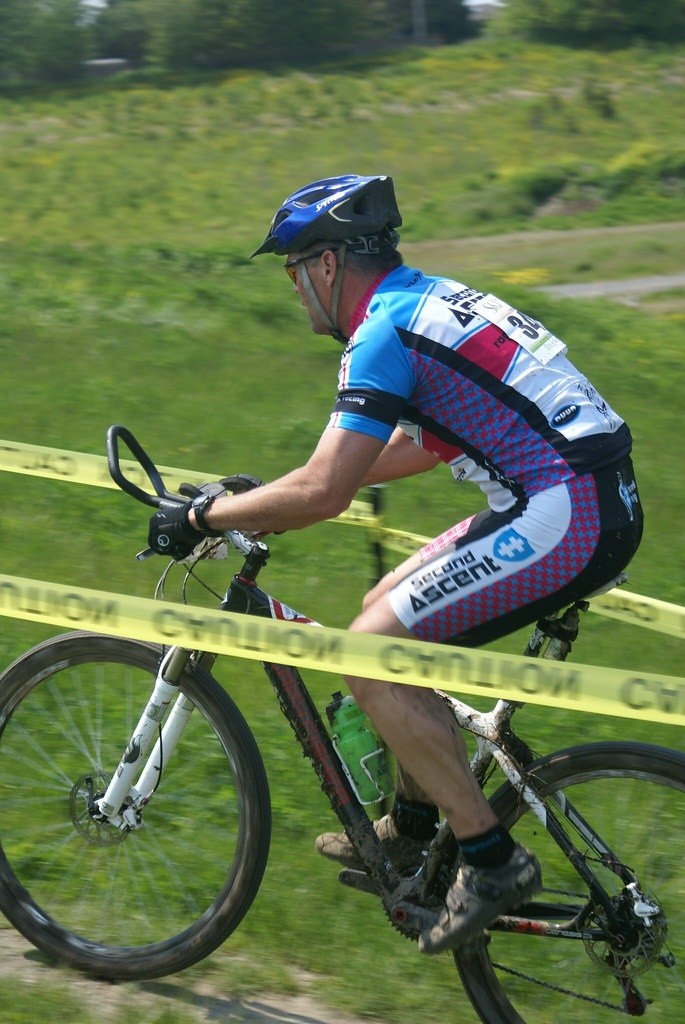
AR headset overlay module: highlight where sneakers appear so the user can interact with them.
[315,814,429,874]
[419,843,542,957]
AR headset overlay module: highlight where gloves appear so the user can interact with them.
[146,502,202,561]
[220,474,286,535]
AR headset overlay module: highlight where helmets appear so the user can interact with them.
[250,174,403,257]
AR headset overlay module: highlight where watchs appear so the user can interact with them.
[191,493,223,538]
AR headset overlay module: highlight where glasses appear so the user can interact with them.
[285,243,340,284]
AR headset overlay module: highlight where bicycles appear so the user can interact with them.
[3,424,685,1024]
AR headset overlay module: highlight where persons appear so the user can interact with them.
[146,172,645,955]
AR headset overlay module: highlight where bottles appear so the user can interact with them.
[324,690,395,802]
